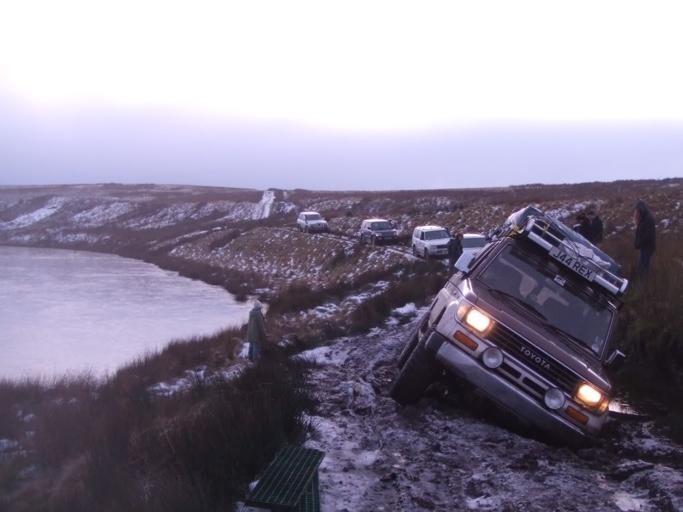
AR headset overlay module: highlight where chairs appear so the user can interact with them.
[540,296,566,322]
[493,262,521,293]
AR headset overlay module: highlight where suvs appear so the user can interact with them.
[359,219,399,248]
[410,226,453,262]
[391,204,630,452]
[296,212,329,234]
[445,232,487,272]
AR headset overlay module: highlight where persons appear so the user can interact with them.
[634,201,656,283]
[446,232,464,276]
[573,211,603,244]
